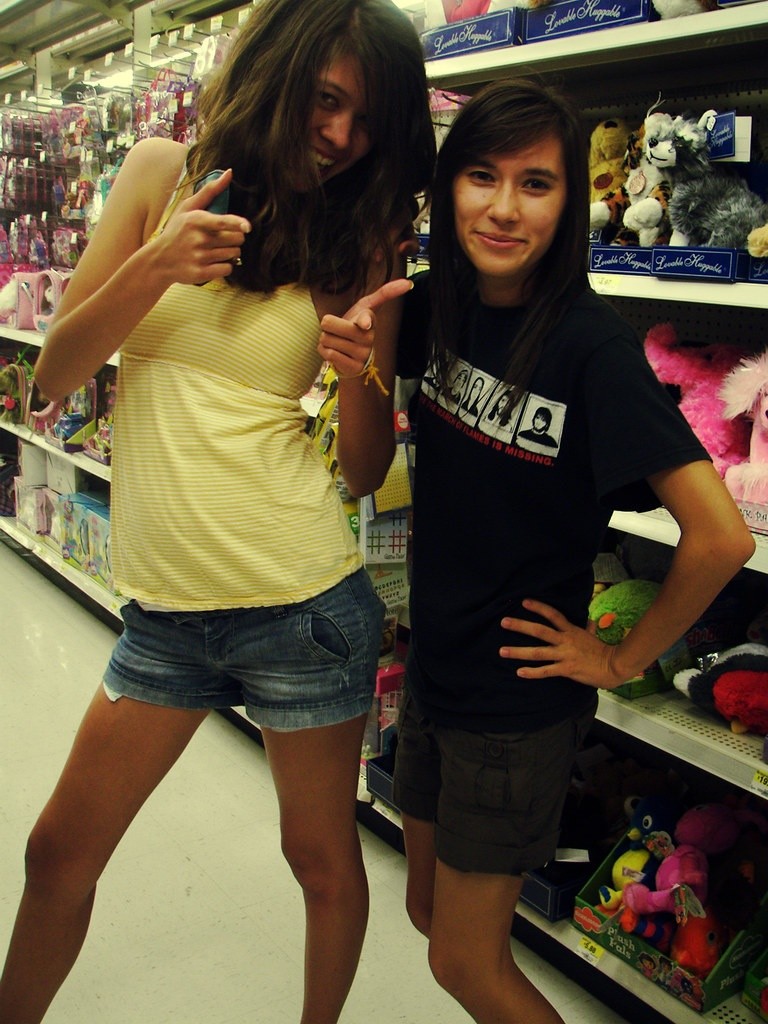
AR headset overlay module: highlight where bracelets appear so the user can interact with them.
[330,346,389,398]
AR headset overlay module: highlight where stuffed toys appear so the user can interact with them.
[588,111,768,980]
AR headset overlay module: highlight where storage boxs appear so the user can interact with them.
[651,249,747,282]
[590,250,655,274]
[522,1,662,41]
[417,9,520,61]
[14,440,123,598]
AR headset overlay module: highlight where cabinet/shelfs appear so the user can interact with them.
[370,0,768,1024]
[0,0,366,810]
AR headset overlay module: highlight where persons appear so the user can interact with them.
[395,80,756,1024]
[0,0,434,1024]
